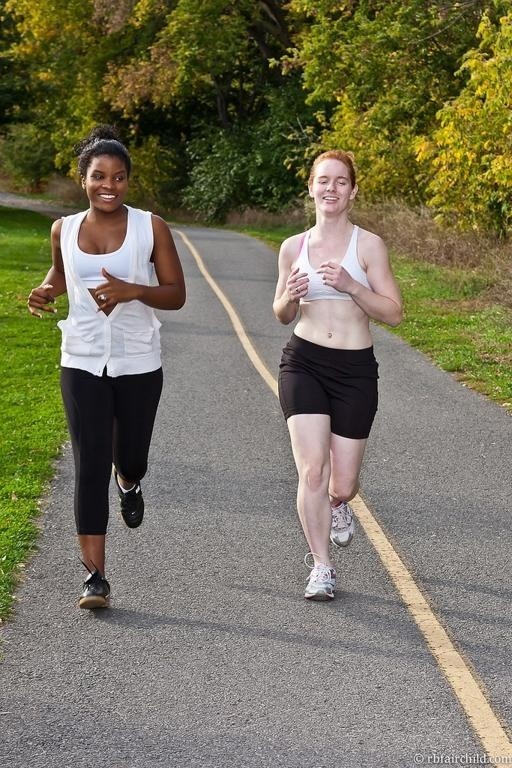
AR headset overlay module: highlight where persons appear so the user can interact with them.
[26,122,186,609]
[273,150,403,601]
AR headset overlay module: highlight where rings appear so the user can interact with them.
[295,288,298,292]
[100,294,106,301]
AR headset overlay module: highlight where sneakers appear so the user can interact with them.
[78,570,111,608]
[112,465,144,528]
[329,501,355,548]
[304,564,337,601]
